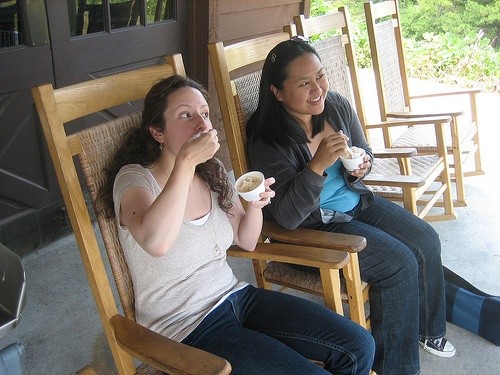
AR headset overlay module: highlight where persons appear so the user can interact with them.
[245,40,456,375]
[90,75,375,375]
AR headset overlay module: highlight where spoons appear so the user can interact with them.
[338,130,359,159]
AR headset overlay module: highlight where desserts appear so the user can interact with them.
[340,146,366,171]
[234,171,265,202]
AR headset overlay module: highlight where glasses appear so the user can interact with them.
[270,35,309,71]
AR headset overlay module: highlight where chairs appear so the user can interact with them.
[364,1,486,208]
[31,53,351,375]
[207,24,426,327]
[293,6,457,223]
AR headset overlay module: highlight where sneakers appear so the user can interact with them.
[418,333,456,358]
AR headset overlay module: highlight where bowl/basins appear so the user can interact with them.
[234,171,266,202]
[340,147,366,172]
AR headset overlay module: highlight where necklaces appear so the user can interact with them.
[188,179,222,256]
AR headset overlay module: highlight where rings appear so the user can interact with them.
[268,200,271,204]
